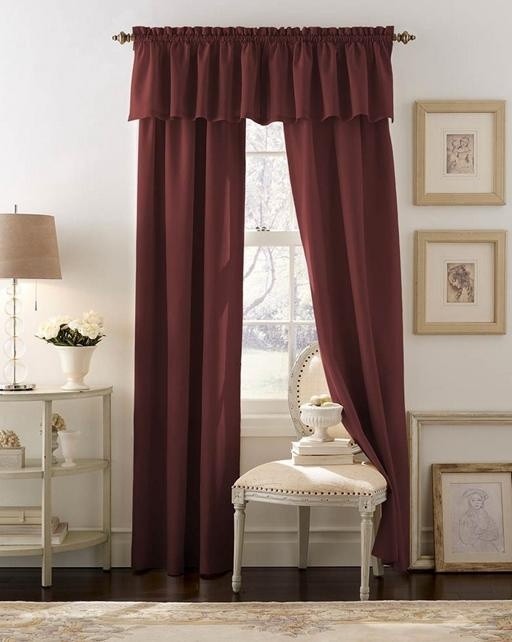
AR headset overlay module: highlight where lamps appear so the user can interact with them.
[1,203,64,394]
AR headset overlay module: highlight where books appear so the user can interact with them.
[0,516,60,534]
[1,522,68,546]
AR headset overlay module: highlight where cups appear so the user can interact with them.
[58,430,80,467]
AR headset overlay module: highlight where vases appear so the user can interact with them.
[52,348,99,392]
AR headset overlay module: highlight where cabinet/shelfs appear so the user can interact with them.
[0,382,114,588]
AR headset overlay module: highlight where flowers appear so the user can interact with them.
[33,308,109,347]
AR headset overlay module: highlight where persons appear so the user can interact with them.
[447,264,474,303]
[446,136,473,174]
[459,488,499,553]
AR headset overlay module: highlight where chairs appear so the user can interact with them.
[229,338,388,601]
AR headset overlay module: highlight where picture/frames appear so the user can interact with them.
[410,96,508,208]
[411,227,509,337]
[428,461,512,575]
